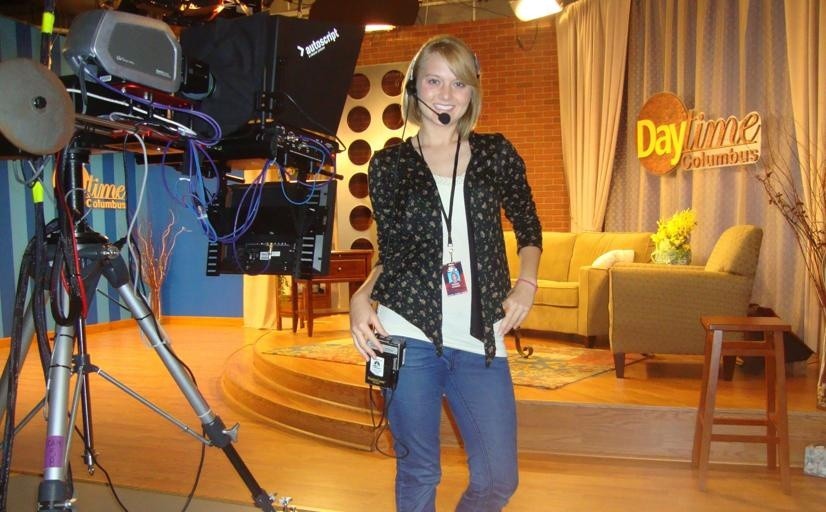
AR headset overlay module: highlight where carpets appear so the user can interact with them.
[255,332,652,393]
[2,467,303,511]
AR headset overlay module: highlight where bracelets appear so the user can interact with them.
[517,279,539,289]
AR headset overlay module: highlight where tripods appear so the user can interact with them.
[0,158,276,512]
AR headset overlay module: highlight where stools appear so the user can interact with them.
[693,316,797,498]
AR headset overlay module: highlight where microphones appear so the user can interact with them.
[408,90,450,125]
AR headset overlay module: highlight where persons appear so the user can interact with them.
[448,272,459,285]
[347,31,545,511]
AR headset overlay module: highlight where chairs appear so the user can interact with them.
[610,225,765,380]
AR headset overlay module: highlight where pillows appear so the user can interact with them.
[590,248,636,272]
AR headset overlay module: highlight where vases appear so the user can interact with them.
[661,252,692,265]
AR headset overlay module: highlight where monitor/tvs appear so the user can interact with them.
[218,180,337,275]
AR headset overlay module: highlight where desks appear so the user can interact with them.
[273,249,376,338]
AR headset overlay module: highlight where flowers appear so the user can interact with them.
[651,206,698,264]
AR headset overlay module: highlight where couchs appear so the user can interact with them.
[502,222,652,348]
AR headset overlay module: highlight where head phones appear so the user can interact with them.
[406,78,417,94]
